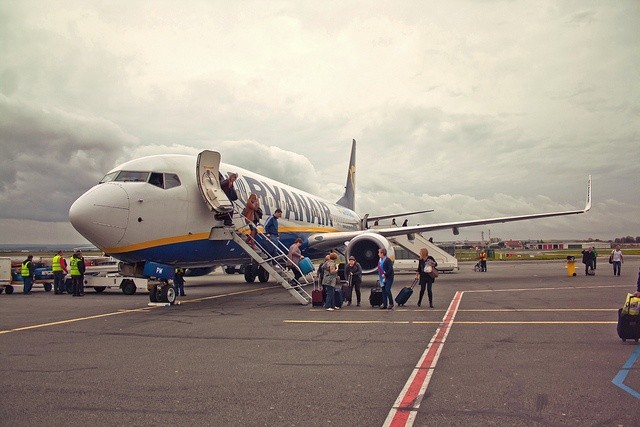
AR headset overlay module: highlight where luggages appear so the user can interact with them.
[334,288,343,307]
[369,280,382,307]
[312,276,323,307]
[298,256,315,276]
[395,279,419,306]
[617,296,640,341]
[342,273,353,302]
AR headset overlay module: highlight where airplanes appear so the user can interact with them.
[68,140,592,306]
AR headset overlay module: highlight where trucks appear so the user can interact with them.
[0,258,14,295]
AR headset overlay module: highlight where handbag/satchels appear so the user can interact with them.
[230,183,237,200]
[609,249,615,264]
[424,263,438,279]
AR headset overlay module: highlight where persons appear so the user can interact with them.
[173,268,186,296]
[414,246,437,309]
[344,256,362,307]
[317,252,340,311]
[52,250,69,295]
[610,244,625,276]
[582,246,598,277]
[70,250,85,297]
[633,266,639,298]
[428,237,433,242]
[392,218,397,226]
[402,219,408,227]
[479,249,487,272]
[378,248,394,309]
[216,173,238,226]
[21,255,34,294]
[374,218,380,226]
[286,237,304,285]
[264,208,283,263]
[244,194,261,249]
